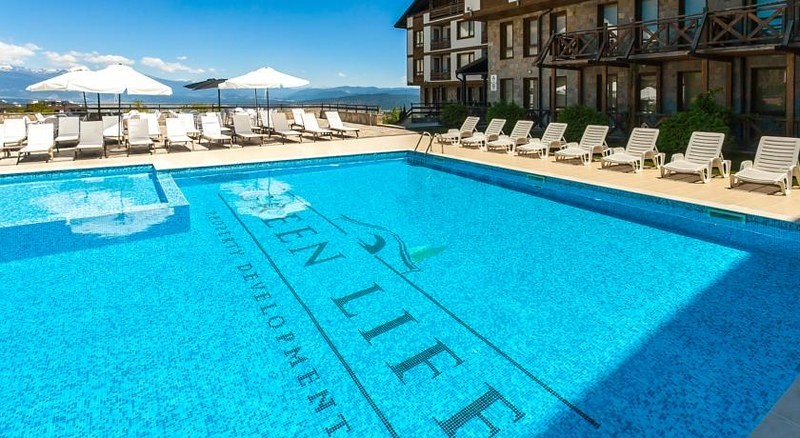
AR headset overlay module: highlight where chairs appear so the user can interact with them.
[601,127,666,174]
[554,124,613,166]
[437,116,481,145]
[731,136,800,195]
[486,120,534,154]
[461,118,509,151]
[0,106,364,167]
[660,131,732,183]
[517,122,567,159]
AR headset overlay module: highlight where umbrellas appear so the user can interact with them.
[67,63,173,152]
[226,66,312,141]
[184,77,230,111]
[26,65,90,121]
[218,67,267,127]
